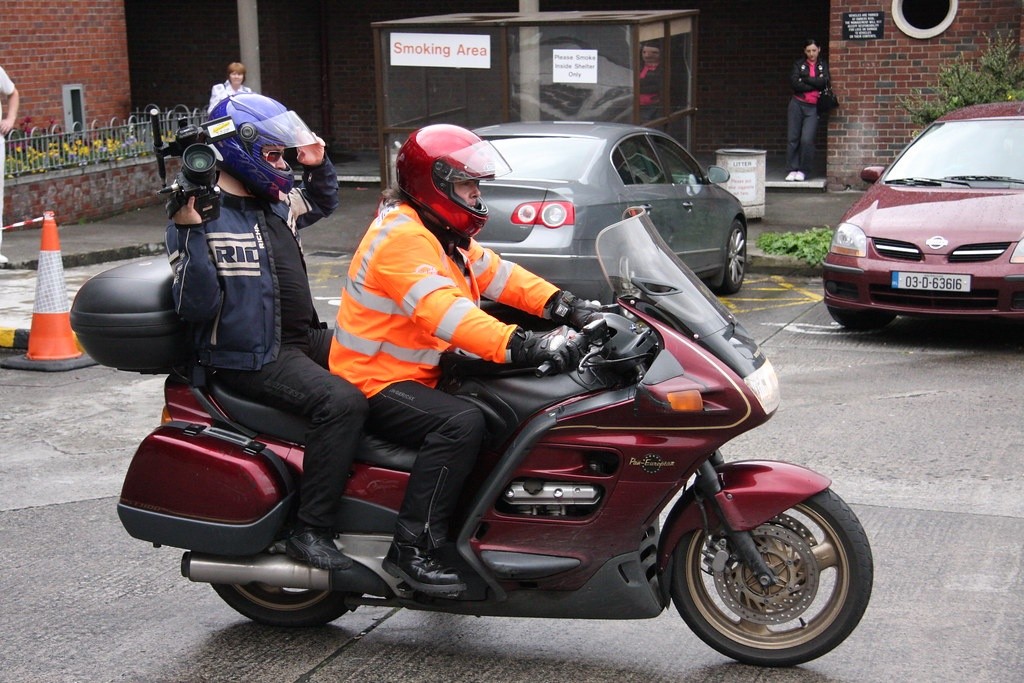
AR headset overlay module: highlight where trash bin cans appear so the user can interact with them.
[714,149,768,222]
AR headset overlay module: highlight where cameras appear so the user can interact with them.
[175,143,222,225]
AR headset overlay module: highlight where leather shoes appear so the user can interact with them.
[382,535,467,593]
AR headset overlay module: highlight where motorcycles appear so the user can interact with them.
[67,204,875,668]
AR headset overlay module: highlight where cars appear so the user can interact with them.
[821,100,1024,344]
[377,121,749,324]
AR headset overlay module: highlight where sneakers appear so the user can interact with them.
[794,170,805,180]
[285,521,353,571]
[785,171,797,181]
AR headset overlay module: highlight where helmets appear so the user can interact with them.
[395,125,495,238]
[206,93,295,194]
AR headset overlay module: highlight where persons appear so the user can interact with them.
[206,63,253,118]
[0,66,20,262]
[328,124,604,592]
[786,40,830,182]
[638,43,666,130]
[163,94,367,569]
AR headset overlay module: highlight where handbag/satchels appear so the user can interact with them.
[817,77,839,114]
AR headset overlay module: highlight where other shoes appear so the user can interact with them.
[0,254,8,263]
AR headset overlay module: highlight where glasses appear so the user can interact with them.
[263,148,285,163]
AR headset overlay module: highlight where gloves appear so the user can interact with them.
[551,290,603,330]
[520,324,588,376]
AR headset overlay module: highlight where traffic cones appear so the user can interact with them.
[0,210,99,373]
